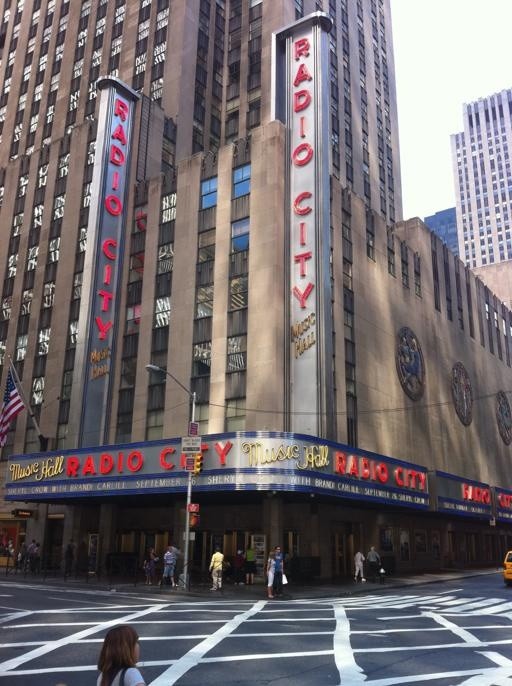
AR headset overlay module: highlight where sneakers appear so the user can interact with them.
[354,577,367,582]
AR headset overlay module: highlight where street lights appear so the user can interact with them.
[145,364,199,594]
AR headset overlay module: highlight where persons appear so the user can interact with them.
[353,552,366,581]
[96,626,146,686]
[367,546,381,582]
[266,552,276,598]
[243,549,257,585]
[209,547,224,590]
[158,546,175,586]
[273,545,283,596]
[144,547,158,585]
[21,539,40,573]
[234,550,245,585]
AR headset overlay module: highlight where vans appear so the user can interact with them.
[503,551,512,585]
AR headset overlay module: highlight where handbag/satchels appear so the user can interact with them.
[281,573,288,585]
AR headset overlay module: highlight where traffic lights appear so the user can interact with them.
[188,513,200,528]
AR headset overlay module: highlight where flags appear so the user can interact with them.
[0,368,25,448]
